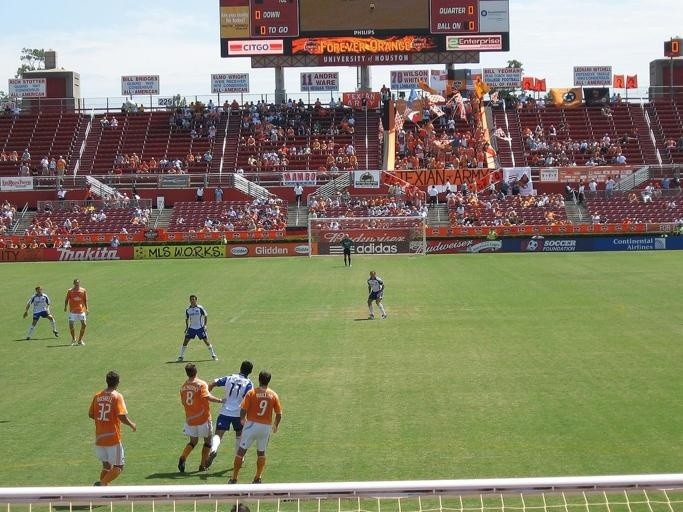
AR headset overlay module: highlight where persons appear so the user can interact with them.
[178,363,227,473]
[367,271,387,320]
[1,85,683,249]
[88,371,136,486]
[231,502,250,512]
[207,361,254,469]
[64,279,89,345]
[228,370,282,484]
[23,286,59,340]
[339,234,354,267]
[176,295,218,361]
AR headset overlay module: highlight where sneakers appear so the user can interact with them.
[228,477,238,484]
[178,455,185,473]
[198,450,217,471]
[252,476,263,484]
[177,355,185,361]
[212,354,219,361]
[26,331,85,346]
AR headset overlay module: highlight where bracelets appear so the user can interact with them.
[219,399,221,403]
